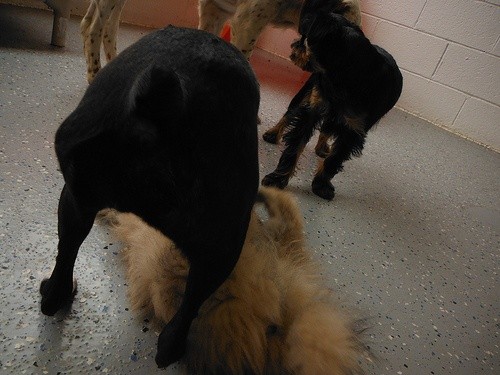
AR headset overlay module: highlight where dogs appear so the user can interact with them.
[261,9,404,202]
[36,26,263,370]
[79,0,363,88]
[96,183,372,374]
